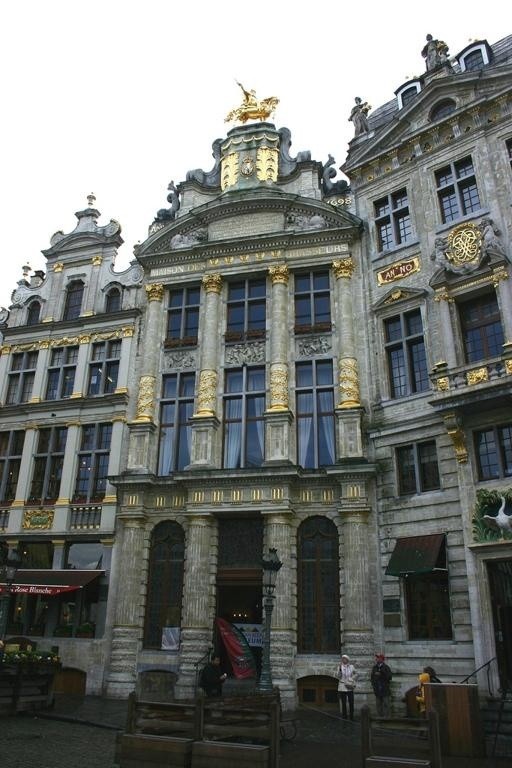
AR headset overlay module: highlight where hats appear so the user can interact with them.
[375,654,384,661]
[342,654,351,662]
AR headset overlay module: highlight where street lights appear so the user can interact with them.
[256,547,284,691]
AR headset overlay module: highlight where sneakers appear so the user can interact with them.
[342,713,353,719]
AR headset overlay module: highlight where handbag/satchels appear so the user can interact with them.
[344,677,356,689]
[198,669,207,687]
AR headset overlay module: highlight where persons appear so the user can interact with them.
[338,654,360,720]
[415,674,429,717]
[202,653,227,698]
[423,666,441,683]
[370,653,393,716]
